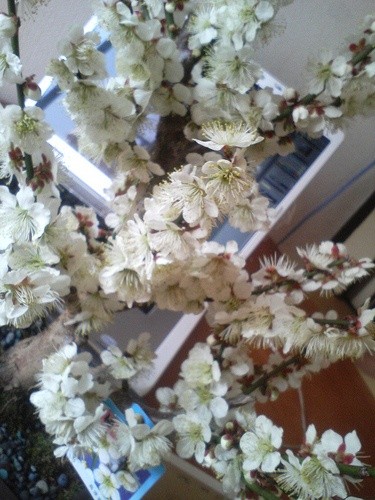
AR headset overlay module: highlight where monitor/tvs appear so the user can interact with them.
[22,1,344,399]
